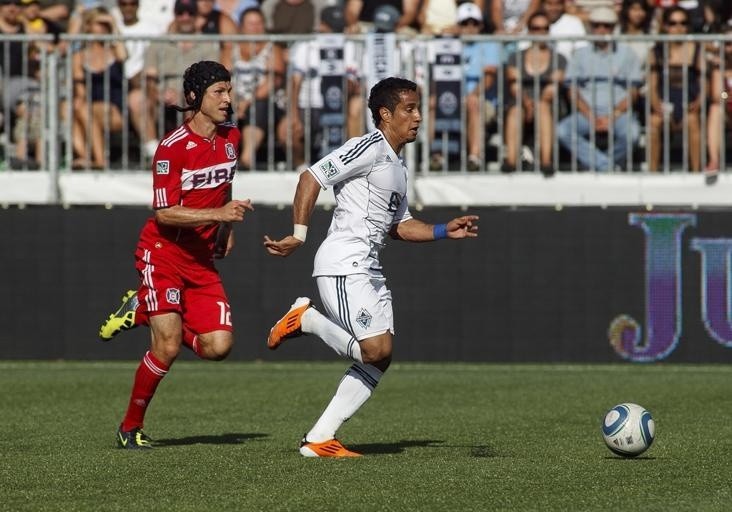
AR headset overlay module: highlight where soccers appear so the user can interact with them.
[603,403,658,456]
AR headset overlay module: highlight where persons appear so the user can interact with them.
[261,76,480,457]
[98,59,255,449]
[0,0,731,184]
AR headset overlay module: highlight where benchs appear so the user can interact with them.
[102,108,689,173]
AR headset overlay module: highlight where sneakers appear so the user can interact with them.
[299,432,365,458]
[116,422,158,450]
[268,297,315,351]
[100,288,140,339]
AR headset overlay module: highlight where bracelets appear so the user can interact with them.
[293,223,308,243]
[433,223,447,241]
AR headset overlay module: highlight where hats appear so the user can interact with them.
[324,8,347,31]
[587,5,616,23]
[456,3,482,24]
[373,8,399,30]
